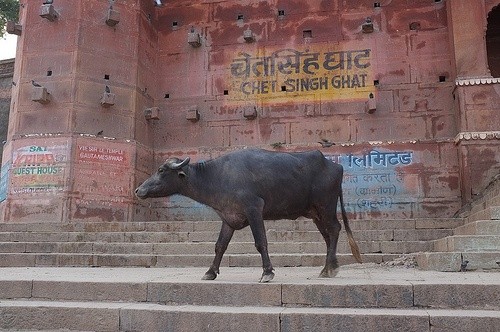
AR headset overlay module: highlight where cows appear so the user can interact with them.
[134,146,362,283]
